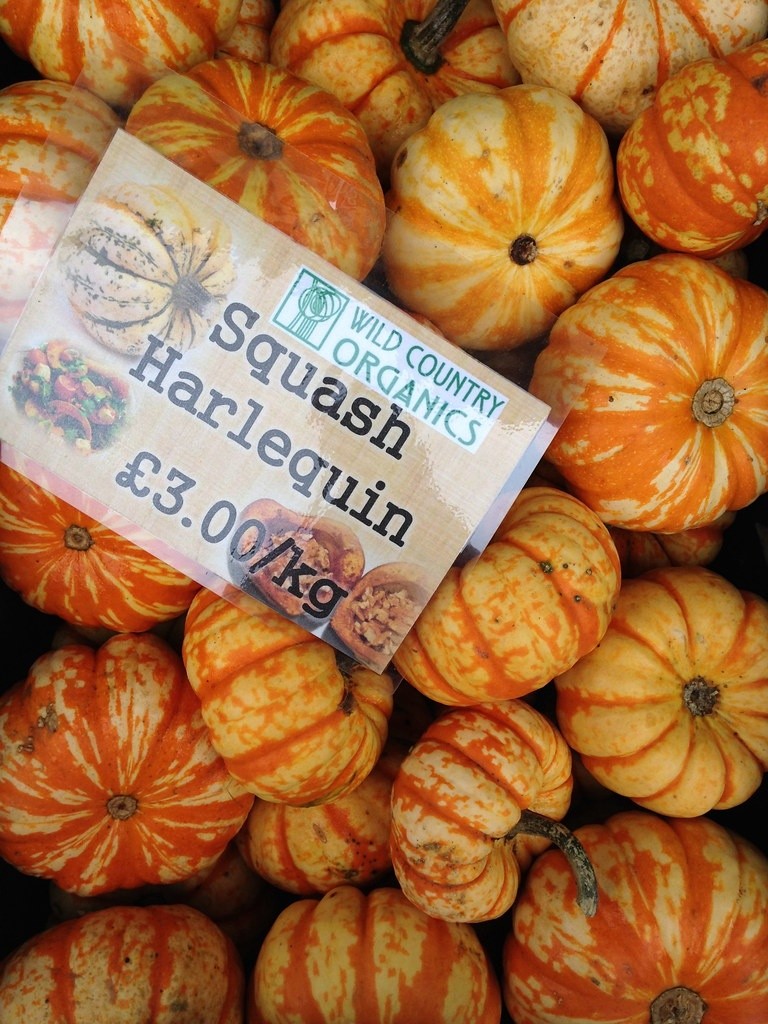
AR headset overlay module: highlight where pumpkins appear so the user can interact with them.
[0,0,768,1019]
